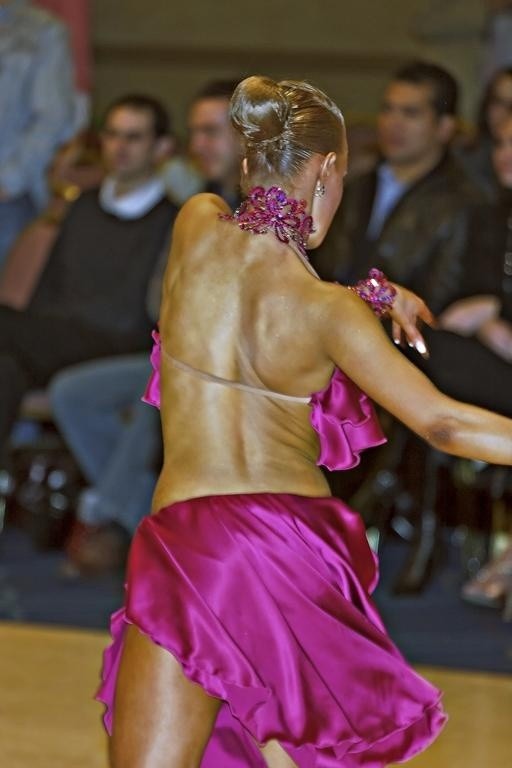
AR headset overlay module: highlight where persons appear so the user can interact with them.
[1,0,511,635]
[88,73,512,768]
